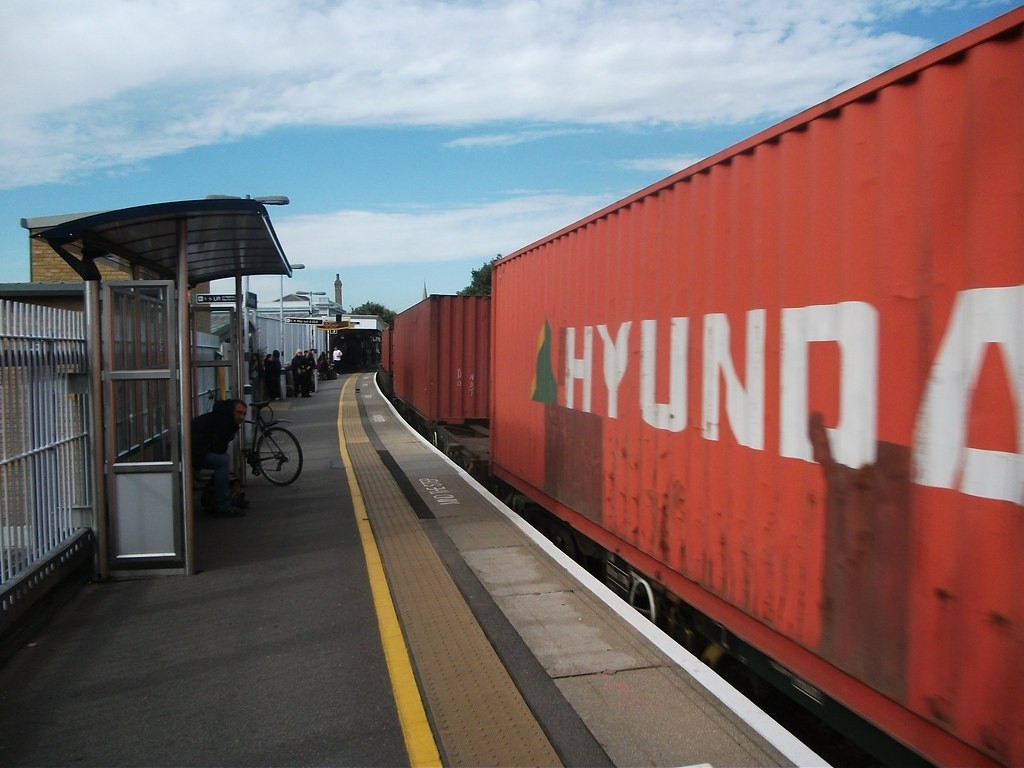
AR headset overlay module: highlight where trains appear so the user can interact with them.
[382,2,1023,767]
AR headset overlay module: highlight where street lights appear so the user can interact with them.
[278,264,305,400]
[295,291,326,351]
[207,194,290,444]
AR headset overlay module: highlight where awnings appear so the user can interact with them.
[28,198,292,289]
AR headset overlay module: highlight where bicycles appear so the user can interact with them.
[206,389,303,486]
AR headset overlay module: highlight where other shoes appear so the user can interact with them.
[207,505,248,516]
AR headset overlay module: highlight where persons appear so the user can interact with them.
[291,349,307,398]
[318,355,329,373]
[301,350,312,398]
[307,351,317,393]
[264,354,273,399]
[310,349,317,393]
[182,398,249,518]
[271,350,282,401]
[322,352,340,376]
[333,346,343,376]
